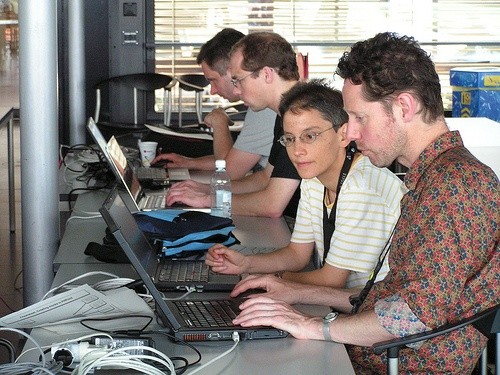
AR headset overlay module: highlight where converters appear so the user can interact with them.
[87,335,157,363]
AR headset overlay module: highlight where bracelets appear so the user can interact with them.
[275,272,285,278]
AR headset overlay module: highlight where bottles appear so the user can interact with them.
[210,160,232,219]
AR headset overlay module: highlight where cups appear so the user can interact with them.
[138,141,158,168]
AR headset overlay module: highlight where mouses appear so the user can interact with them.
[149,160,173,168]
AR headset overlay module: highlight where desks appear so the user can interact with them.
[53,192,314,272]
[0,106,16,233]
[57,149,254,200]
[15,263,356,375]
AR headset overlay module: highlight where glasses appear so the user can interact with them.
[278,123,344,147]
[231,65,273,88]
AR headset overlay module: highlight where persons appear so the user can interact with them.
[150,28,277,180]
[231,32,500,375]
[204,78,406,289]
[167,32,301,229]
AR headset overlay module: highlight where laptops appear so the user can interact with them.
[86,117,189,182]
[98,186,241,291]
[110,225,291,341]
[105,135,185,210]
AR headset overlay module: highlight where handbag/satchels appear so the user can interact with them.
[134,210,240,258]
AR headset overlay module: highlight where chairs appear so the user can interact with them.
[373,304,500,375]
[94,73,211,151]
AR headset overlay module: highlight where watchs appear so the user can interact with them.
[323,312,339,342]
